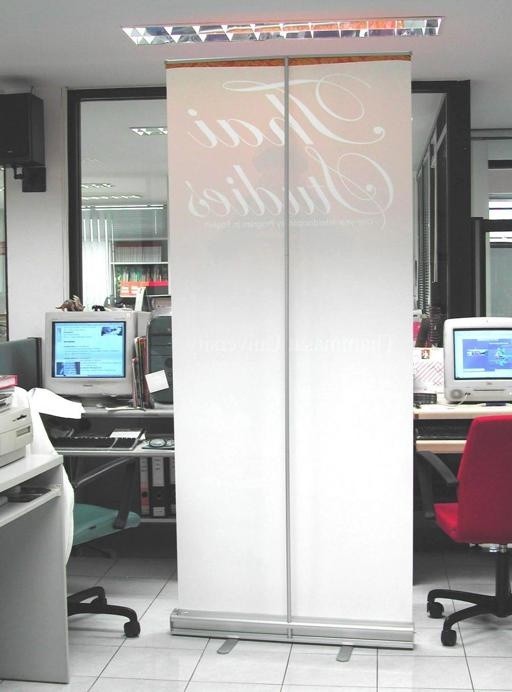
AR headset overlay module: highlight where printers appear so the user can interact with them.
[0,379,34,469]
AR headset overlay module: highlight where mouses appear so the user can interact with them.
[149,437,166,447]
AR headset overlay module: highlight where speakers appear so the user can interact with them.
[0,93,45,167]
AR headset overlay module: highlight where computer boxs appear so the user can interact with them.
[148,316,173,405]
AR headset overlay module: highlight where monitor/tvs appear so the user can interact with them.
[43,310,138,407]
[443,317,512,407]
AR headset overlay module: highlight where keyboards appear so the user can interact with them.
[415,419,470,439]
[51,435,139,451]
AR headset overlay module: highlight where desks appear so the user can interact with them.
[413,400,512,584]
[0,453,72,685]
[43,398,175,557]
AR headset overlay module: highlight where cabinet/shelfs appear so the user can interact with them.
[108,239,172,317]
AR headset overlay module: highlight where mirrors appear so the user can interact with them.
[65,78,473,345]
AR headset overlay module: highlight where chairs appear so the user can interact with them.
[426,414,512,645]
[67,458,141,637]
